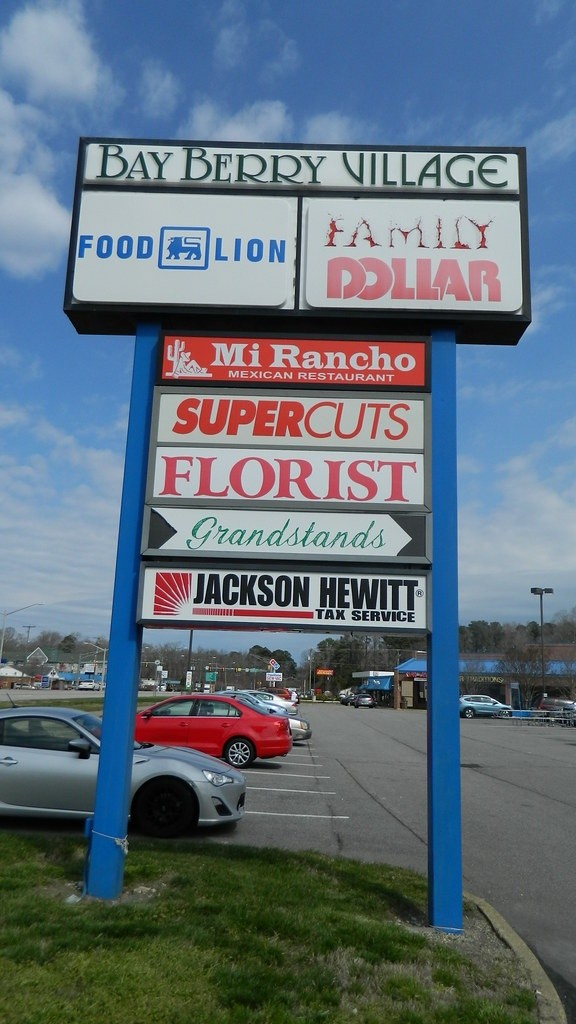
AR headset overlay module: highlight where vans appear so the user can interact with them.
[538,698,576,711]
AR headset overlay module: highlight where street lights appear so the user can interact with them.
[83,641,109,683]
[0,602,46,666]
[531,588,554,693]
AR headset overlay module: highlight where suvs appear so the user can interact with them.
[459,695,512,719]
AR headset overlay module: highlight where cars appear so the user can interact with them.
[78,679,101,691]
[0,707,247,838]
[355,694,374,708]
[135,687,312,768]
